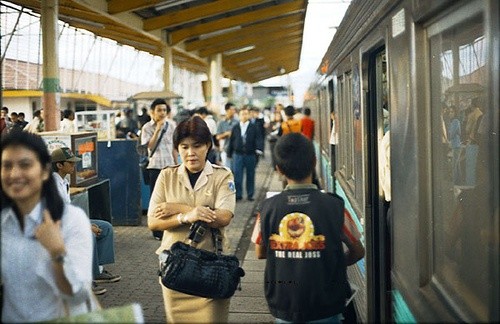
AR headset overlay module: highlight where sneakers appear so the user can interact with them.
[248,197,255,201]
[93,283,107,295]
[236,196,242,201]
[96,268,121,283]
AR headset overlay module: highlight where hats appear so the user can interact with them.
[50,147,83,163]
[284,106,297,116]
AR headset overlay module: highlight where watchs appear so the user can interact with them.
[53,254,67,265]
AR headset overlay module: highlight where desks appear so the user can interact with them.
[70,181,113,227]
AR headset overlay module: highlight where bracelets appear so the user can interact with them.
[176,214,182,228]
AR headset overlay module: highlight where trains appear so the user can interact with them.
[302,0,499,324]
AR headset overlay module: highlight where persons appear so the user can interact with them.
[1,102,78,134]
[51,148,121,294]
[116,107,149,137]
[176,104,216,134]
[250,133,366,324]
[440,95,484,146]
[216,102,265,201]
[147,117,237,323]
[141,99,178,240]
[0,134,101,324]
[265,104,314,170]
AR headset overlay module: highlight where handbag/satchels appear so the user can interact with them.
[141,160,151,185]
[213,135,219,146]
[156,241,244,299]
